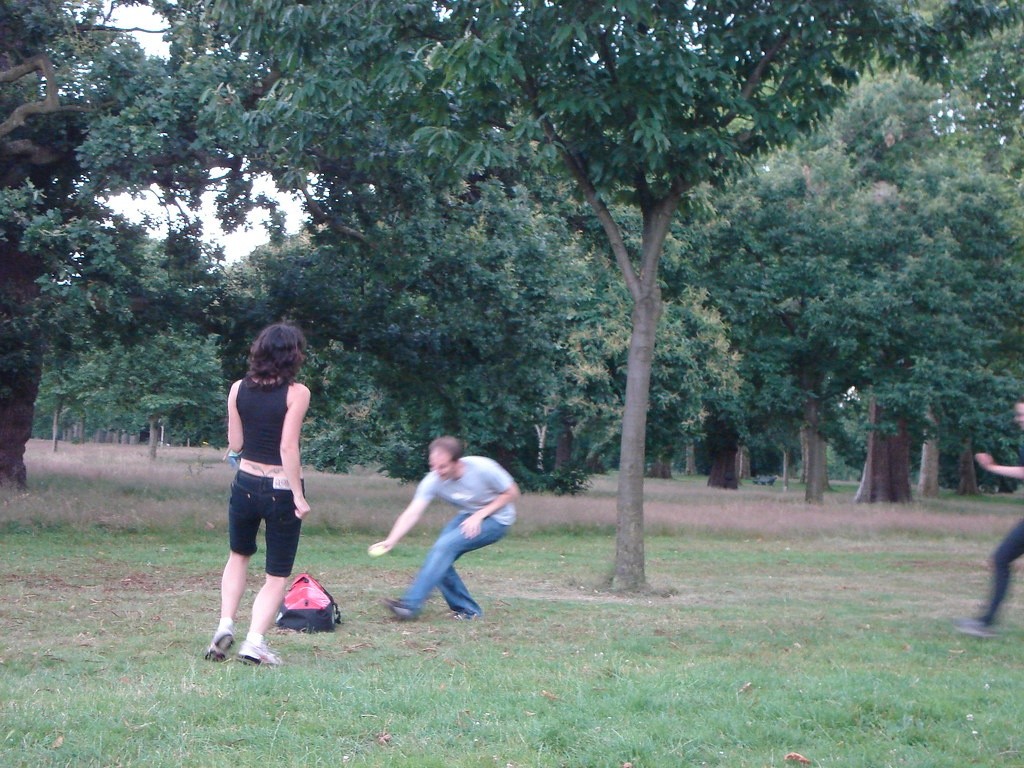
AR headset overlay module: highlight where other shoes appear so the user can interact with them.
[381,598,419,621]
[441,613,470,622]
[956,619,997,637]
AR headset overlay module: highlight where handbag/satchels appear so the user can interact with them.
[276,573,341,633]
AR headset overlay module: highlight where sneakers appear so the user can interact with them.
[205,630,235,662]
[237,641,283,667]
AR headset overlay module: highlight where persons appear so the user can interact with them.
[368,434,522,620]
[204,324,311,664]
[954,400,1024,637]
